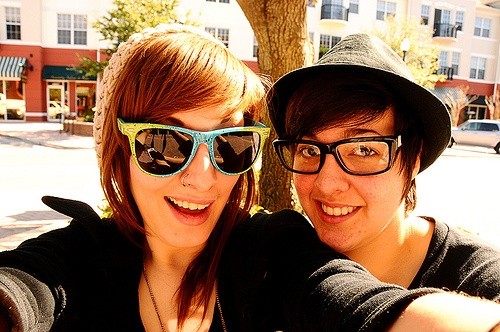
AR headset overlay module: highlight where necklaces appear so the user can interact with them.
[143,264,227,332]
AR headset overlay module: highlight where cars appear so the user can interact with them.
[448,119,500,154]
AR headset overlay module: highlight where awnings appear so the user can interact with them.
[0,56,27,81]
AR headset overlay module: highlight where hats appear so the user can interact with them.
[265,34,452,174]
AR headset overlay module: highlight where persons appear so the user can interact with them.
[134,129,194,169]
[215,135,238,164]
[0,23,500,332]
[265,32,500,305]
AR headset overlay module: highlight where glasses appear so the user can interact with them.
[273,126,412,176]
[116,117,271,177]
